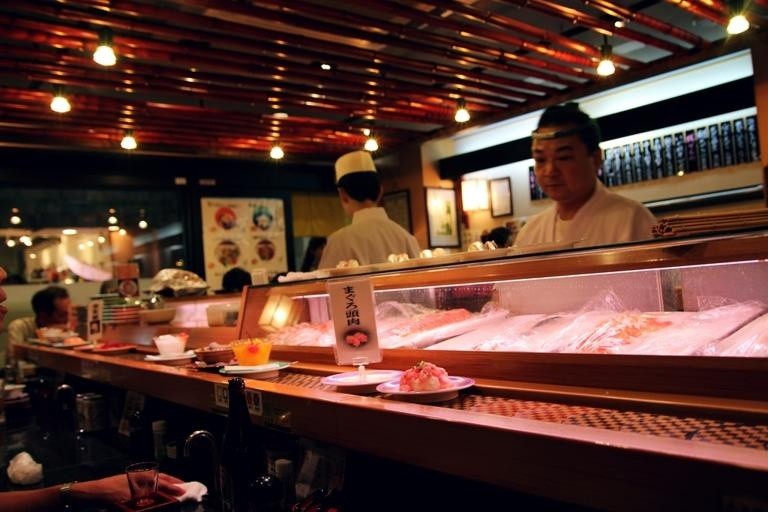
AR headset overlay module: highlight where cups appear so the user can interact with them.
[125,461,160,509]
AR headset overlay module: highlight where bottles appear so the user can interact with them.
[151,418,177,459]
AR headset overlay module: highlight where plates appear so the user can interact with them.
[24,295,292,382]
[321,369,406,393]
[376,375,475,403]
[323,238,578,277]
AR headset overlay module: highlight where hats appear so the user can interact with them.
[334,151,377,184]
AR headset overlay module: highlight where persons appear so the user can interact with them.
[316,171,421,270]
[511,101,660,249]
[7,285,77,367]
[222,266,253,292]
[300,236,327,272]
[0,265,187,511]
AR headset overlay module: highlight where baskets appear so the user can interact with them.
[434,284,494,311]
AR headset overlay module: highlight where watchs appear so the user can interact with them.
[59,479,75,510]
